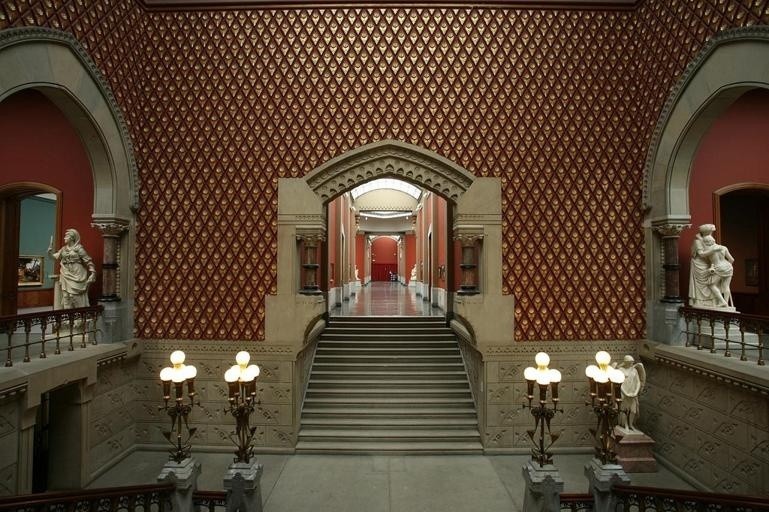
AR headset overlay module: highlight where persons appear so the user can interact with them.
[615,355,647,431]
[48,228,96,319]
[688,223,734,307]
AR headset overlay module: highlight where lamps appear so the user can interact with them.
[158,349,197,465]
[519,347,562,467]
[219,350,261,465]
[580,350,624,466]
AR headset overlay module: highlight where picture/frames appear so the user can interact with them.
[744,258,758,287]
[17,255,45,287]
